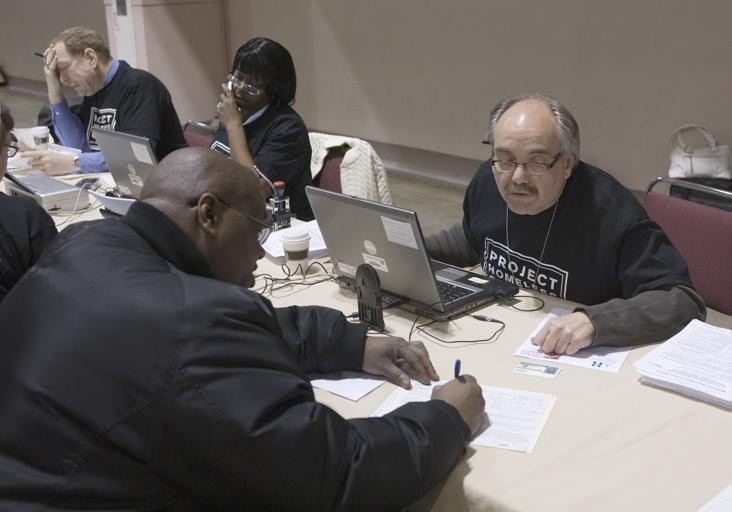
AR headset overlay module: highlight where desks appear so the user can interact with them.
[670,178,732,211]
[0,140,732,512]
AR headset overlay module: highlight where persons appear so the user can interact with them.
[19,25,189,176]
[1,146,486,512]
[422,93,707,356]
[208,36,315,221]
[0,99,59,300]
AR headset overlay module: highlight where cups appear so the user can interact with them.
[281,229,310,275]
[32,126,49,150]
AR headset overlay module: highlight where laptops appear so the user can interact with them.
[90,124,158,194]
[304,183,520,324]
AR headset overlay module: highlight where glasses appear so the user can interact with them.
[487,151,561,175]
[191,195,272,244]
[0,142,19,158]
[226,72,265,96]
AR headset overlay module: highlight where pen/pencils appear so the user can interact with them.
[33,52,44,58]
[455,360,460,377]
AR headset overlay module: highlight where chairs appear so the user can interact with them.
[308,133,392,207]
[643,176,732,317]
[183,120,217,148]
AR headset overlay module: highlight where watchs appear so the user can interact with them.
[73,156,80,168]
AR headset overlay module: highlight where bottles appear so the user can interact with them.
[270,182,291,230]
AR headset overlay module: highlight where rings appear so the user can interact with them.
[46,63,49,65]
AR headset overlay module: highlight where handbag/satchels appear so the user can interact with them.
[667,144,731,180]
